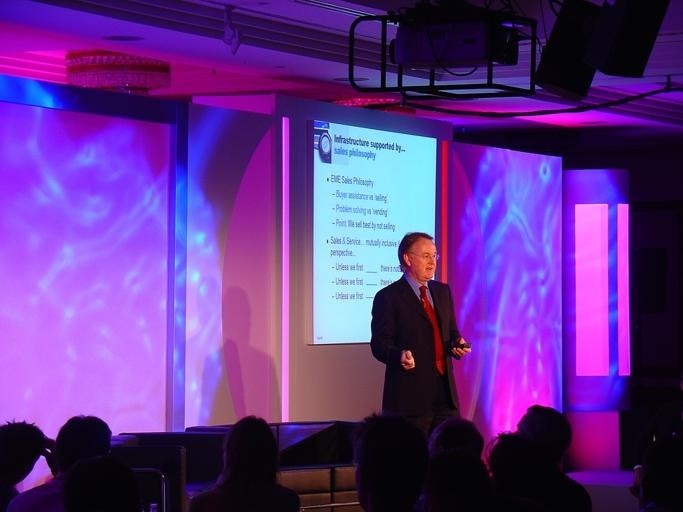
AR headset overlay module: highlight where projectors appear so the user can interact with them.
[389,13,518,68]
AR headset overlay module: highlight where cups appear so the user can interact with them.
[632,461,643,485]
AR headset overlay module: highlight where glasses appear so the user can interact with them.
[405,249,439,262]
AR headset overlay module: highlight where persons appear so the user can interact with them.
[187,416,300,512]
[0,419,56,512]
[632,398,683,512]
[370,233,472,438]
[7,414,140,512]
[352,404,592,512]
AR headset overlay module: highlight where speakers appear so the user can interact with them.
[598,0,671,78]
[534,0,612,100]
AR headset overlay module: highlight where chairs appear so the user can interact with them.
[69,417,366,512]
[630,429,683,512]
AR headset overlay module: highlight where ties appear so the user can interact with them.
[415,286,450,377]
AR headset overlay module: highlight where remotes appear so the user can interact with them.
[449,343,471,351]
